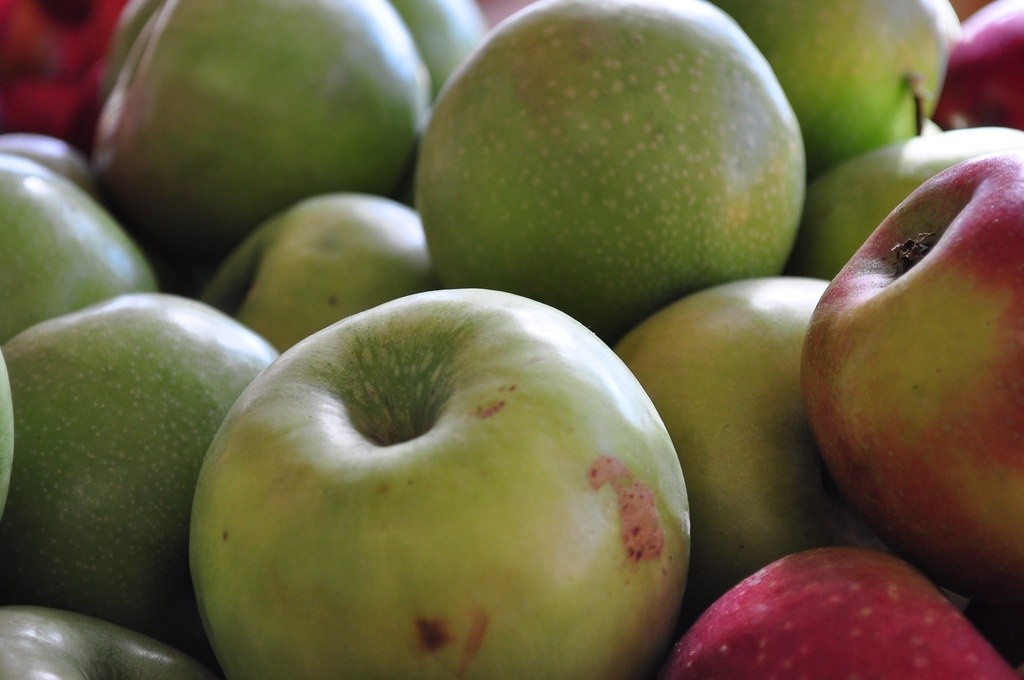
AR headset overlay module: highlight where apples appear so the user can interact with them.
[0,0,1024,680]
